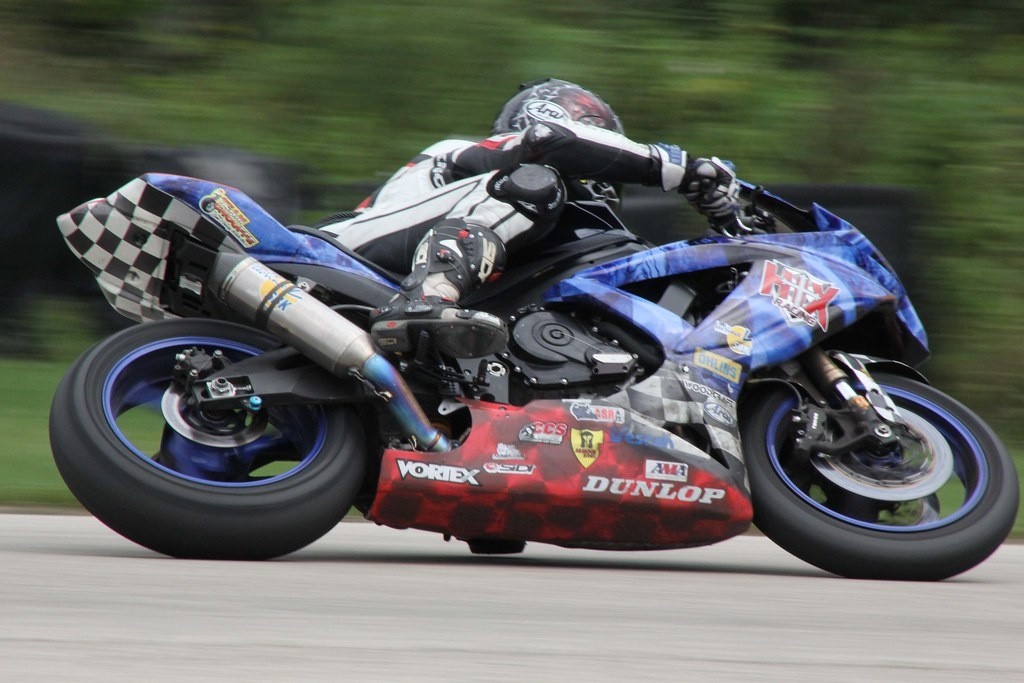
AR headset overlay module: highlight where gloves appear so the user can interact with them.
[644,142,740,229]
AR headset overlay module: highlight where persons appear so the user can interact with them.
[345,77,741,357]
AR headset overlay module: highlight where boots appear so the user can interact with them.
[368,218,509,359]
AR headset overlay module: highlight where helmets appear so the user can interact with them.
[489,77,627,210]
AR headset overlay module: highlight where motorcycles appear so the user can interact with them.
[49,153,1020,583]
